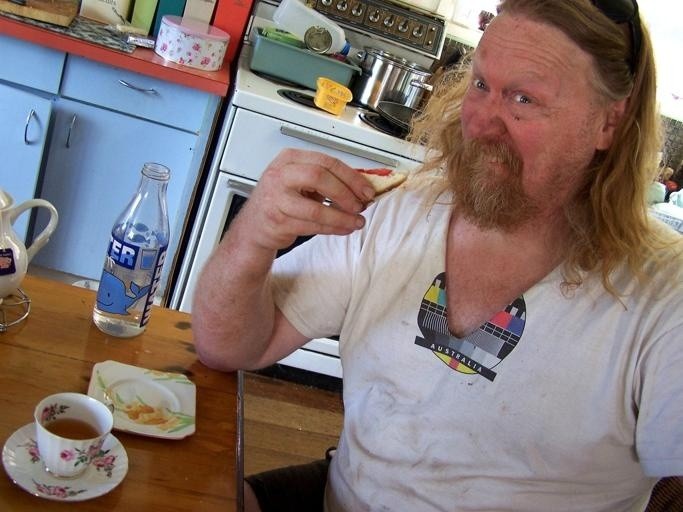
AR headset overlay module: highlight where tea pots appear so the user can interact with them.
[0,187,59,300]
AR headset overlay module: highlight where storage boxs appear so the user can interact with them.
[250,27,362,92]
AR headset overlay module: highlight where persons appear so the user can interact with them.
[187,2,682,512]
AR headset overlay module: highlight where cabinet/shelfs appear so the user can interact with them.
[0,34,222,305]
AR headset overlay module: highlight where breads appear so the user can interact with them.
[355,166,410,214]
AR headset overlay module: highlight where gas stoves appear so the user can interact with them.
[231,44,447,171]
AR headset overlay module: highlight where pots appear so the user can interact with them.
[350,46,433,114]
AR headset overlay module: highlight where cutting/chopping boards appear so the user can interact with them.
[0,0,80,27]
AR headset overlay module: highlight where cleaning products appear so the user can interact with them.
[273,1,367,62]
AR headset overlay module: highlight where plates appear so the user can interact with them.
[1,421,130,503]
[86,358,197,441]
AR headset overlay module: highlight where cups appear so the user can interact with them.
[34,391,114,479]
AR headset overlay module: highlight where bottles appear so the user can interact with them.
[272,0,350,54]
[92,161,171,338]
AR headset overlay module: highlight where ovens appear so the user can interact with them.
[177,170,341,359]
